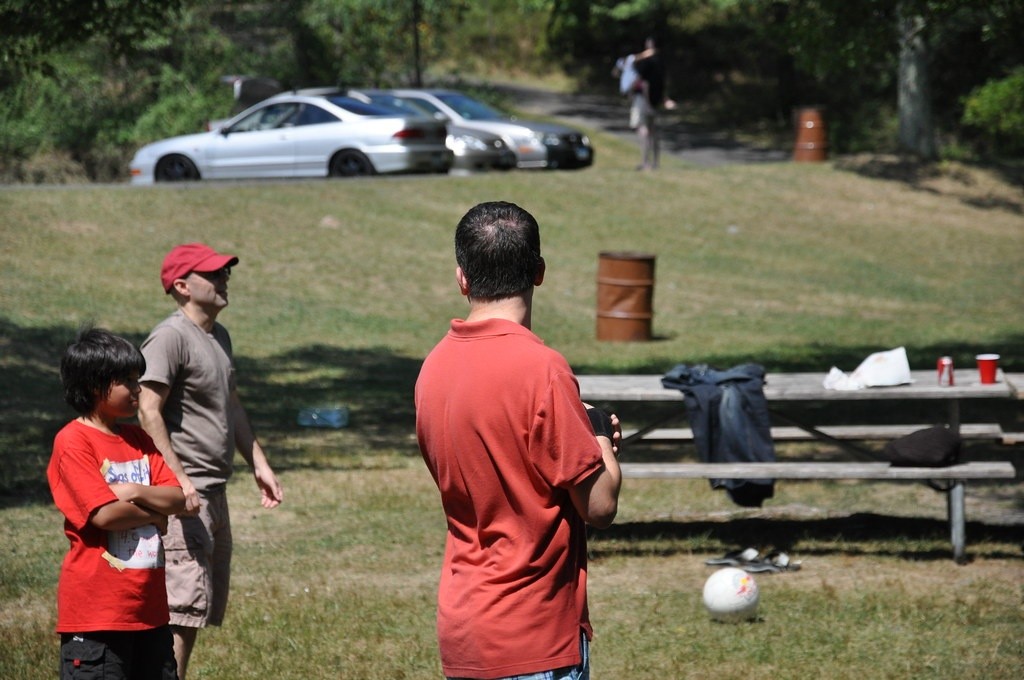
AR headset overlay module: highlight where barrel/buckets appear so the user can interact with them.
[596,251,656,342]
[792,105,827,163]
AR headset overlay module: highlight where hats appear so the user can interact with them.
[162,244,240,295]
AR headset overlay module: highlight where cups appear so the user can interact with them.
[976,354,1000,384]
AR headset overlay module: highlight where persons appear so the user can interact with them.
[132,242,282,680]
[615,39,676,170]
[45,328,186,680]
[414,201,623,680]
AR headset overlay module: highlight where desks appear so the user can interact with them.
[570,366,1023,563]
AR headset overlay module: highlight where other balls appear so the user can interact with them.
[702,565,758,625]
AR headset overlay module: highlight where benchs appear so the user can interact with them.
[595,421,1023,563]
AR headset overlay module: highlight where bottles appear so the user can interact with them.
[297,407,365,429]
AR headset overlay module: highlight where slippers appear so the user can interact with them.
[705,544,802,574]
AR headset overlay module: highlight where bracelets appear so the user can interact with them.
[587,407,616,447]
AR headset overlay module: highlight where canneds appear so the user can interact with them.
[937,356,953,386]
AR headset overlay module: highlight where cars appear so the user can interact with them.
[129,86,595,181]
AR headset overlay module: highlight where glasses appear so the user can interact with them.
[191,266,232,279]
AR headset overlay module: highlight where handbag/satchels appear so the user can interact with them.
[887,427,959,493]
